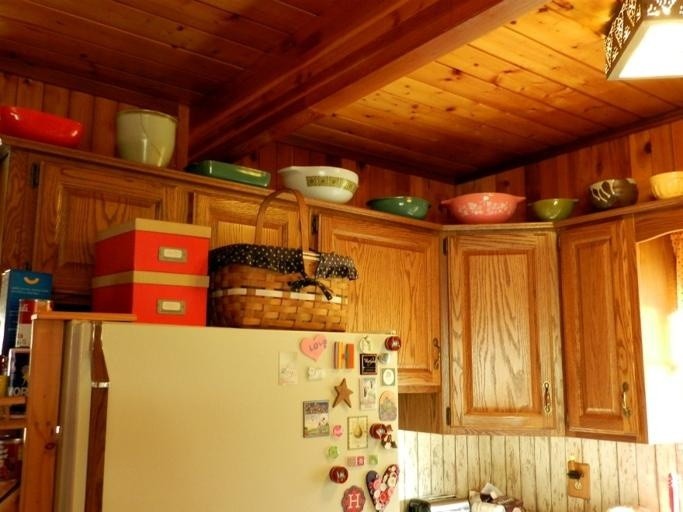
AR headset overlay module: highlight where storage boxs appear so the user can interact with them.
[88,216,214,275]
[85,272,210,328]
[0,266,53,358]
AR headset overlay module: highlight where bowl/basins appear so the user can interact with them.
[588,177,639,211]
[649,171,683,199]
[0,103,84,146]
[440,192,526,224]
[533,199,578,221]
[366,195,433,220]
[278,165,359,205]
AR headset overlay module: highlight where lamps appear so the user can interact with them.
[600,0,683,83]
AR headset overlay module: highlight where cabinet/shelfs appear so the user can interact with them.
[0,311,64,511]
[554,193,682,446]
[312,199,443,395]
[30,141,309,328]
[399,221,568,435]
[0,134,44,301]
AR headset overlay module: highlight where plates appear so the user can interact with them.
[186,159,271,188]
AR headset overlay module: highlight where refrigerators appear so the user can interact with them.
[53,320,400,512]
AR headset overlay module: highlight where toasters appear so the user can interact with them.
[409,494,470,512]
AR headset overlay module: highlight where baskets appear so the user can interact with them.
[207,186,360,334]
